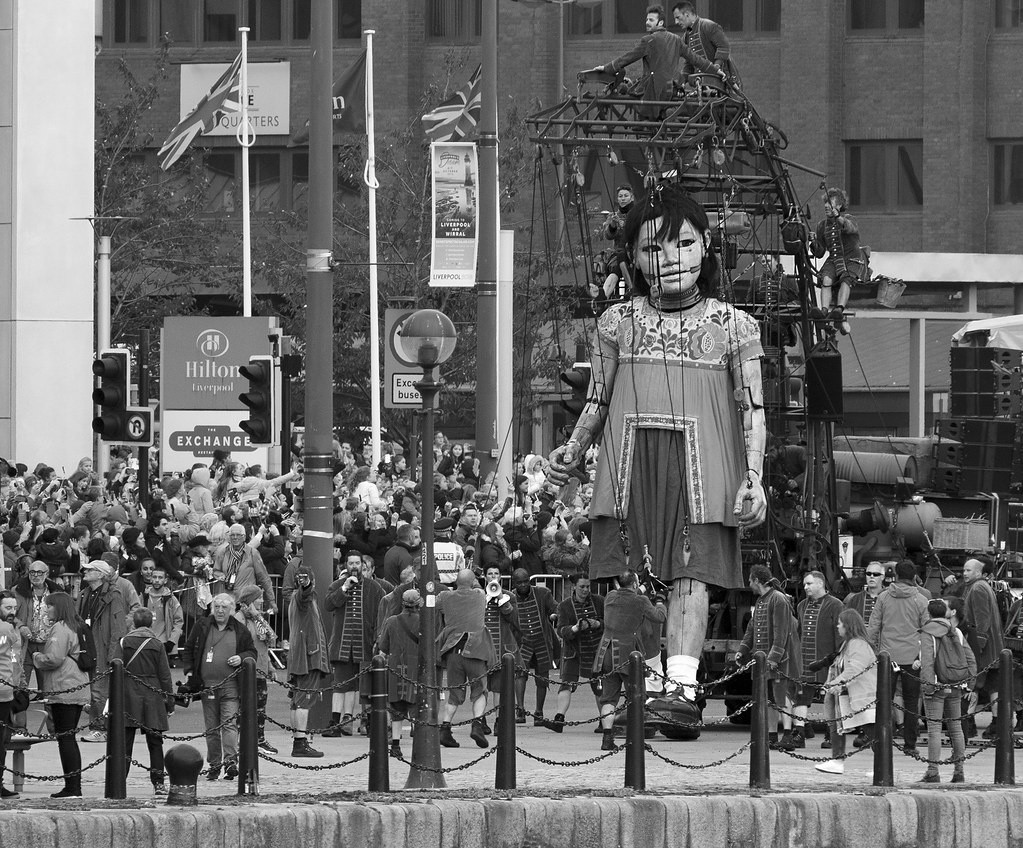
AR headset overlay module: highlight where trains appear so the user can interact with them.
[690,440,995,726]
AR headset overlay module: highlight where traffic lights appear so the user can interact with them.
[238,355,276,448]
[91,349,131,445]
[559,362,594,439]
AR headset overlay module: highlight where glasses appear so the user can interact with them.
[28,569,48,576]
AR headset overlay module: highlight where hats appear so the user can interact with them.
[123,528,142,547]
[535,512,554,531]
[3,531,20,547]
[434,517,456,532]
[188,536,213,548]
[83,560,111,576]
[165,481,181,498]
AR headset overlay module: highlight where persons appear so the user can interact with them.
[547,186,768,730]
[593,184,637,308]
[596,4,728,131]
[1,431,1008,802]
[746,252,799,370]
[671,1,732,87]
[808,187,863,327]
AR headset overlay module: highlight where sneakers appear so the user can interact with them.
[81,730,107,742]
[323,720,342,737]
[515,709,527,723]
[599,732,618,751]
[257,740,278,755]
[224,764,239,780]
[916,772,941,784]
[206,766,220,780]
[594,720,604,734]
[768,733,778,748]
[821,730,832,748]
[968,718,978,736]
[292,737,324,758]
[952,773,966,783]
[154,783,170,795]
[390,743,403,758]
[828,308,844,322]
[853,728,881,747]
[813,306,827,321]
[543,715,564,732]
[336,715,354,735]
[533,711,543,726]
[19,792,31,801]
[945,720,974,738]
[805,723,815,738]
[814,759,844,775]
[1,785,20,799]
[50,787,82,798]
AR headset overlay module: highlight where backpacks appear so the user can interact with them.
[76,621,97,670]
[930,621,970,683]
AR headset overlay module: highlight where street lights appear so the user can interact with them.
[399,310,457,789]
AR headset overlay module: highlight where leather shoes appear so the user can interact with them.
[643,691,702,729]
[614,711,655,739]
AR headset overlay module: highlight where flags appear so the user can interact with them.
[420,62,482,142]
[287,47,367,148]
[156,51,242,172]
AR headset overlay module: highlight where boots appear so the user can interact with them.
[982,716,998,739]
[471,721,488,749]
[438,721,460,747]
[775,730,794,751]
[792,725,806,749]
